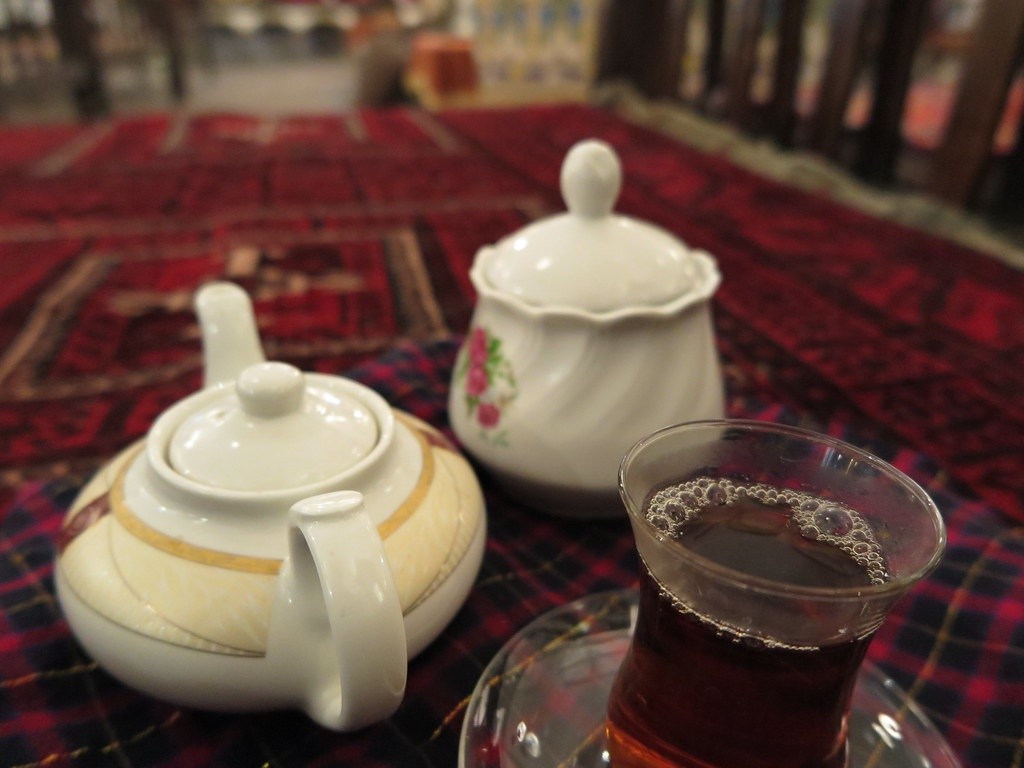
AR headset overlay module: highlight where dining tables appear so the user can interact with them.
[0,96,1024,768]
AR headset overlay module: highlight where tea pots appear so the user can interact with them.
[48,279,492,732]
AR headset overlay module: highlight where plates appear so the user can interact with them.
[456,592,965,767]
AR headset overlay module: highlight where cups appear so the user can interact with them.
[601,420,945,767]
[449,141,730,521]
